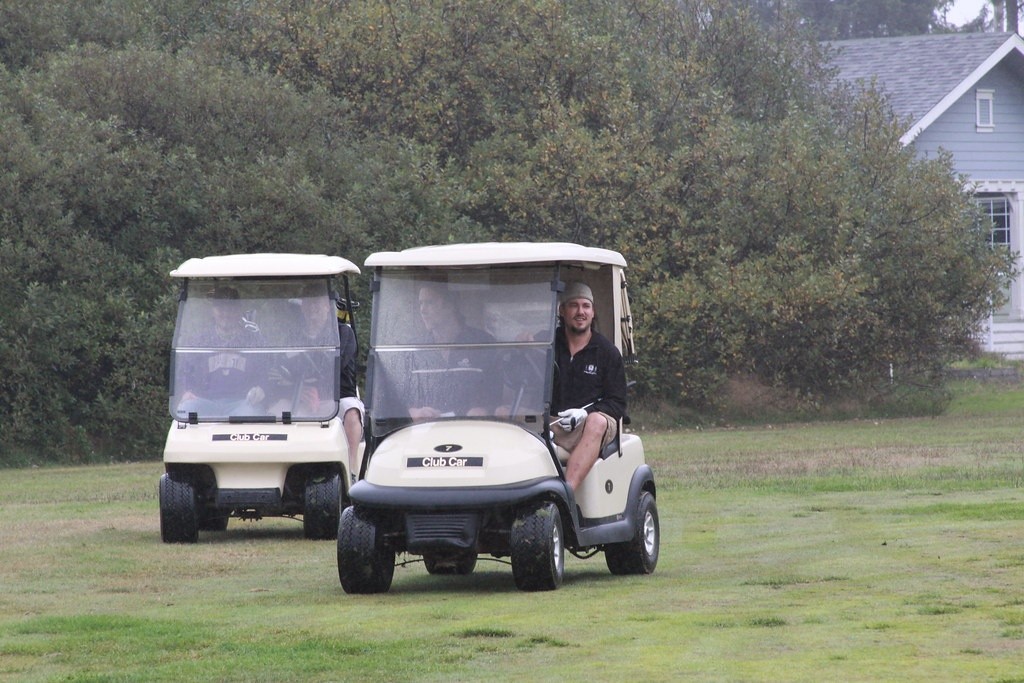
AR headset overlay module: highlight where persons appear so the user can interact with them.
[176,287,280,418]
[380,276,506,421]
[268,284,365,484]
[501,283,627,494]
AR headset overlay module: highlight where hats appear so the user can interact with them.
[211,286,241,306]
[560,282,594,307]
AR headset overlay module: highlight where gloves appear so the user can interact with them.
[557,408,588,433]
